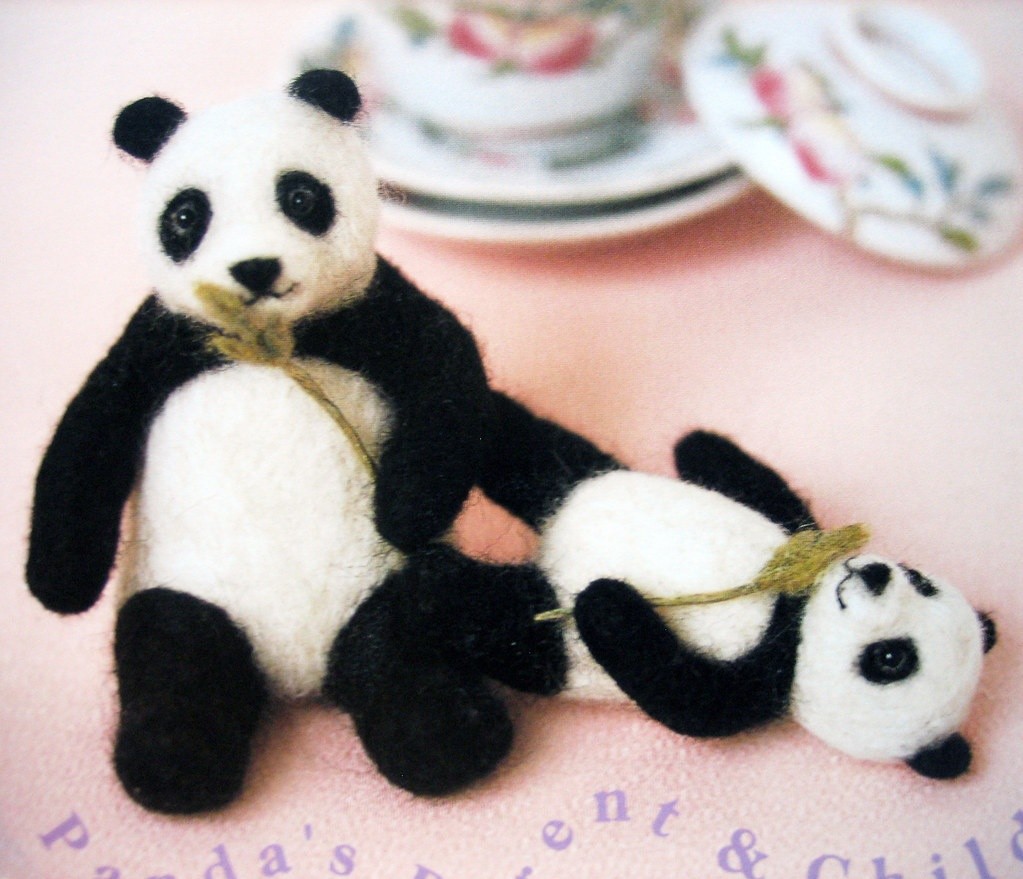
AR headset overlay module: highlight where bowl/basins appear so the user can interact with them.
[682,0,1023,274]
[354,0,675,166]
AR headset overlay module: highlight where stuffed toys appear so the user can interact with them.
[25,70,514,818]
[406,384,999,782]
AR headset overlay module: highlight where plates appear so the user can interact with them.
[352,173,759,244]
[361,113,739,209]
